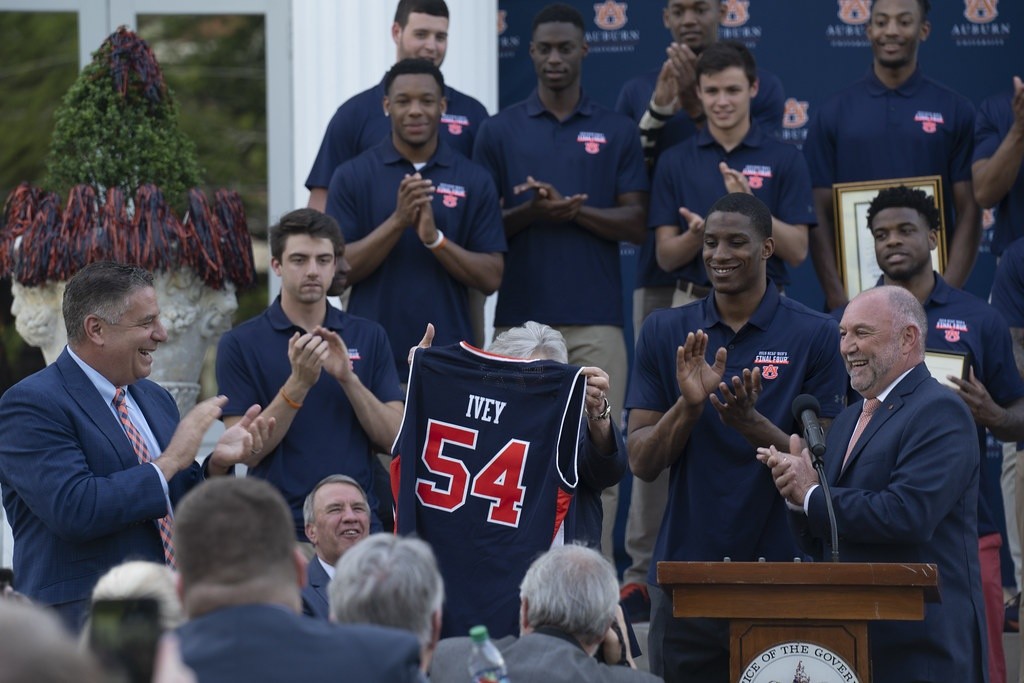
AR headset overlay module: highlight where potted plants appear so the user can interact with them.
[2,24,254,422]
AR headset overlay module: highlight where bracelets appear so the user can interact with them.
[423,229,447,254]
[279,385,304,409]
[585,397,612,420]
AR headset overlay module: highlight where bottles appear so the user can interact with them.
[467,624,510,683]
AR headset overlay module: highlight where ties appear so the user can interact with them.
[115,387,176,571]
[840,399,880,477]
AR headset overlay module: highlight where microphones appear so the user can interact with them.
[792,394,826,456]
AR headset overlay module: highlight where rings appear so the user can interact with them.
[251,448,262,457]
[783,457,788,461]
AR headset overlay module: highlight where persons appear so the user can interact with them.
[971,75,1024,262]
[828,186,1024,683]
[751,284,990,683]
[983,240,1024,633]
[320,57,510,405]
[473,4,649,577]
[625,195,852,683]
[0,474,668,683]
[215,208,407,557]
[0,259,275,656]
[305,0,498,353]
[612,1,986,353]
[611,38,814,632]
[411,320,629,568]
